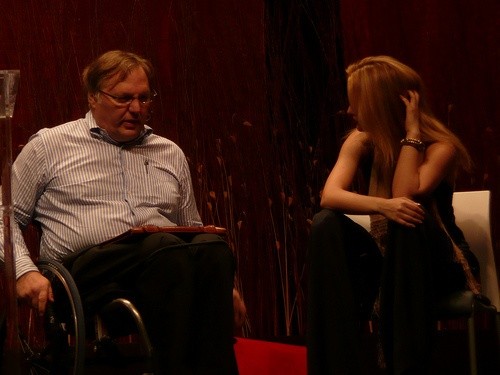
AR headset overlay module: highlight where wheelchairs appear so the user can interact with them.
[13,258,154,375]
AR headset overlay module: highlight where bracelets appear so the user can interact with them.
[400,137,425,153]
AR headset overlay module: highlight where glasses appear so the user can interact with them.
[96,88,158,104]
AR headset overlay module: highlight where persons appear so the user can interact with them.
[0,50,247,375]
[307,56,480,375]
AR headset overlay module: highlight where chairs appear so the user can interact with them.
[345,190,500,375]
